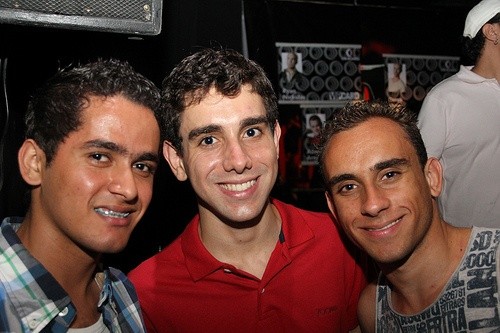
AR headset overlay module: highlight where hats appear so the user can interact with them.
[463,0,500,39]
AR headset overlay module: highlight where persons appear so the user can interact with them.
[387,63,407,111]
[315,101,499,333]
[304,115,324,159]
[280,52,303,92]
[127,46,366,333]
[415,0,499,229]
[0,58,166,333]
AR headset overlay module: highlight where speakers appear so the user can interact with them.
[0,0,163,36]
